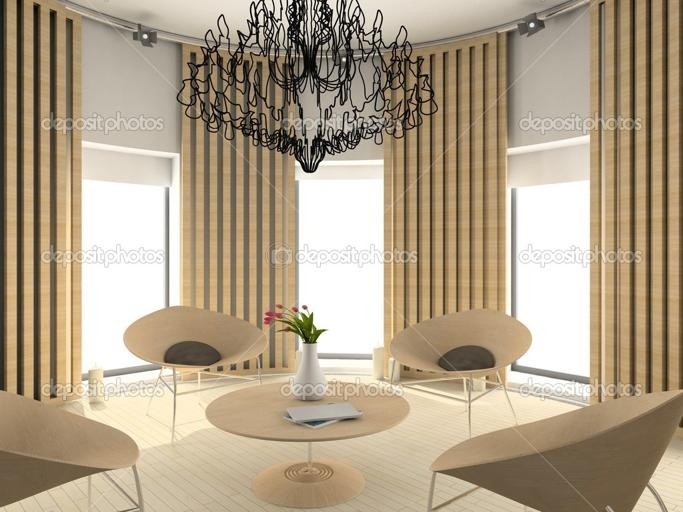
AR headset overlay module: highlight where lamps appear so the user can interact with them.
[516,12,545,38]
[133,0,438,174]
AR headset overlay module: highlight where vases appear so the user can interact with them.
[290,344,328,400]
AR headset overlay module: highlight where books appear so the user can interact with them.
[282,401,363,430]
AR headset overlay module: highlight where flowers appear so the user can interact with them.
[263,302,327,343]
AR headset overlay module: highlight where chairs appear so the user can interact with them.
[0,305,271,511]
[388,307,682,512]
